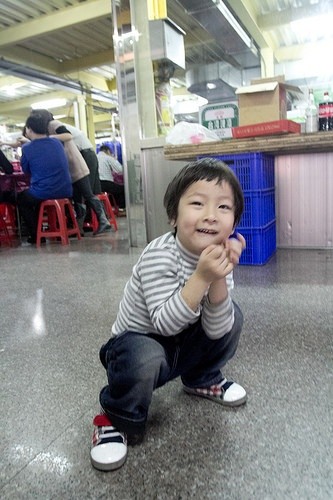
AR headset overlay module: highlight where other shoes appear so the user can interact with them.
[26,236,47,243]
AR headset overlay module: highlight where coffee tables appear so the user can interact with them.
[84,192,119,233]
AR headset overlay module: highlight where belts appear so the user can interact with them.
[81,148,90,155]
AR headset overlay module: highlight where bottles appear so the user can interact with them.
[319,92,333,131]
[307,88,318,132]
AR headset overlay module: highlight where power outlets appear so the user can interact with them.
[235,75,304,126]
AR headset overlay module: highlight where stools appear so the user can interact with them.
[0,203,20,249]
[36,198,81,246]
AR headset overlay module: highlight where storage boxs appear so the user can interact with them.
[197,152,277,266]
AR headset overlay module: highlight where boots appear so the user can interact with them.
[69,203,87,238]
[88,195,112,234]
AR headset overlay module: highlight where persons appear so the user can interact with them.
[31,109,110,238]
[19,113,72,247]
[91,158,249,473]
[0,149,18,205]
[98,145,126,217]
[1,139,19,150]
[61,122,101,233]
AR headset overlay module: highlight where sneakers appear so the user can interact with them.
[88,414,127,472]
[183,377,247,407]
